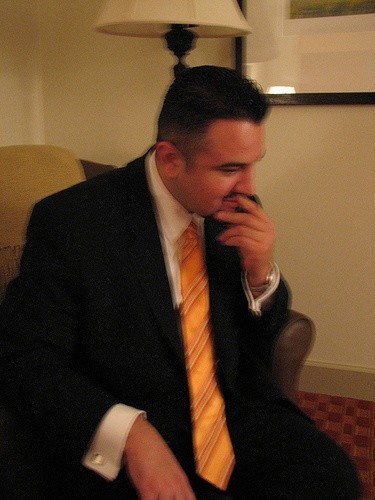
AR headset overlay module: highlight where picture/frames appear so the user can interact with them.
[232,0,375,108]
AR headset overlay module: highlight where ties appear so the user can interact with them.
[180,215,238,491]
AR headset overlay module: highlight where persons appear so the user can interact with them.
[7,65,367,500]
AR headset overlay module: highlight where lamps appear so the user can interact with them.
[88,0,256,86]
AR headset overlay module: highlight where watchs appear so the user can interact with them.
[248,266,276,294]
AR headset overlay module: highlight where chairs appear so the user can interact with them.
[0,140,317,414]
